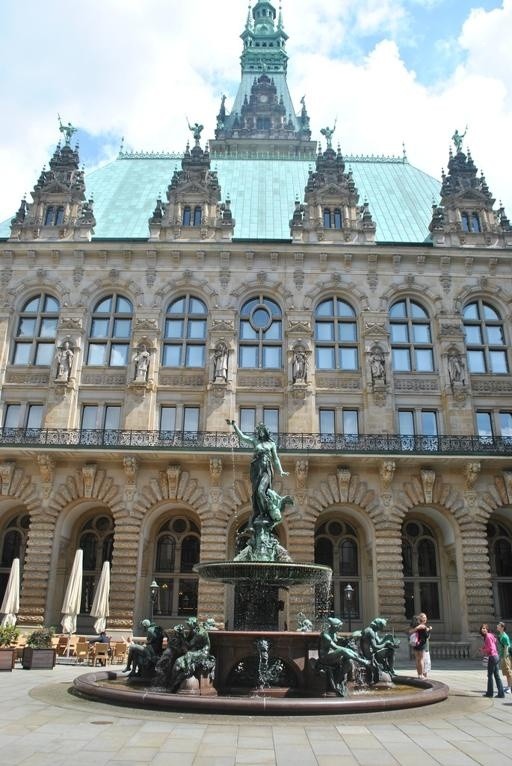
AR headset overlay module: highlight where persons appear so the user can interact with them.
[408,616,432,679]
[413,613,431,679]
[222,417,294,531]
[476,624,504,699]
[358,619,399,670]
[120,620,164,677]
[491,622,511,696]
[122,636,135,665]
[164,618,213,694]
[88,632,113,666]
[315,617,375,676]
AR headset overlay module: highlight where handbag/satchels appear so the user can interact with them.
[509,647,512,658]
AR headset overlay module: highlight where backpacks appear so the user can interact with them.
[409,629,423,648]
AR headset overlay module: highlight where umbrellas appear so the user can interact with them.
[0,557,22,627]
[58,548,86,664]
[89,560,111,635]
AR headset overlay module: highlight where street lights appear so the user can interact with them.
[150,580,159,623]
[344,583,355,632]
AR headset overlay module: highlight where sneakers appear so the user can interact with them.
[495,695,504,698]
[503,687,512,694]
[483,693,493,697]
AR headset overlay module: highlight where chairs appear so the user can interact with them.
[0,630,132,671]
